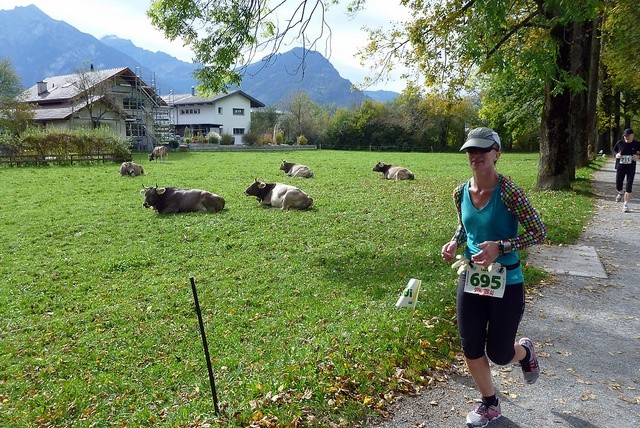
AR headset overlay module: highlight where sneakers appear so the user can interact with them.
[466,398,501,427]
[616,190,626,202]
[623,204,628,212]
[519,338,540,384]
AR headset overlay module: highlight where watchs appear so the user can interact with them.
[500,238,512,254]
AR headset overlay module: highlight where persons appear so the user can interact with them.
[612,128,640,212]
[440,126,546,426]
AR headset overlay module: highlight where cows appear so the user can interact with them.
[243,177,313,212]
[139,182,225,217]
[278,158,314,178]
[372,159,415,181]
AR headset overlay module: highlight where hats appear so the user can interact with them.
[623,128,633,136]
[460,127,501,152]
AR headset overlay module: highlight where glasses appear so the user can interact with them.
[465,147,498,153]
